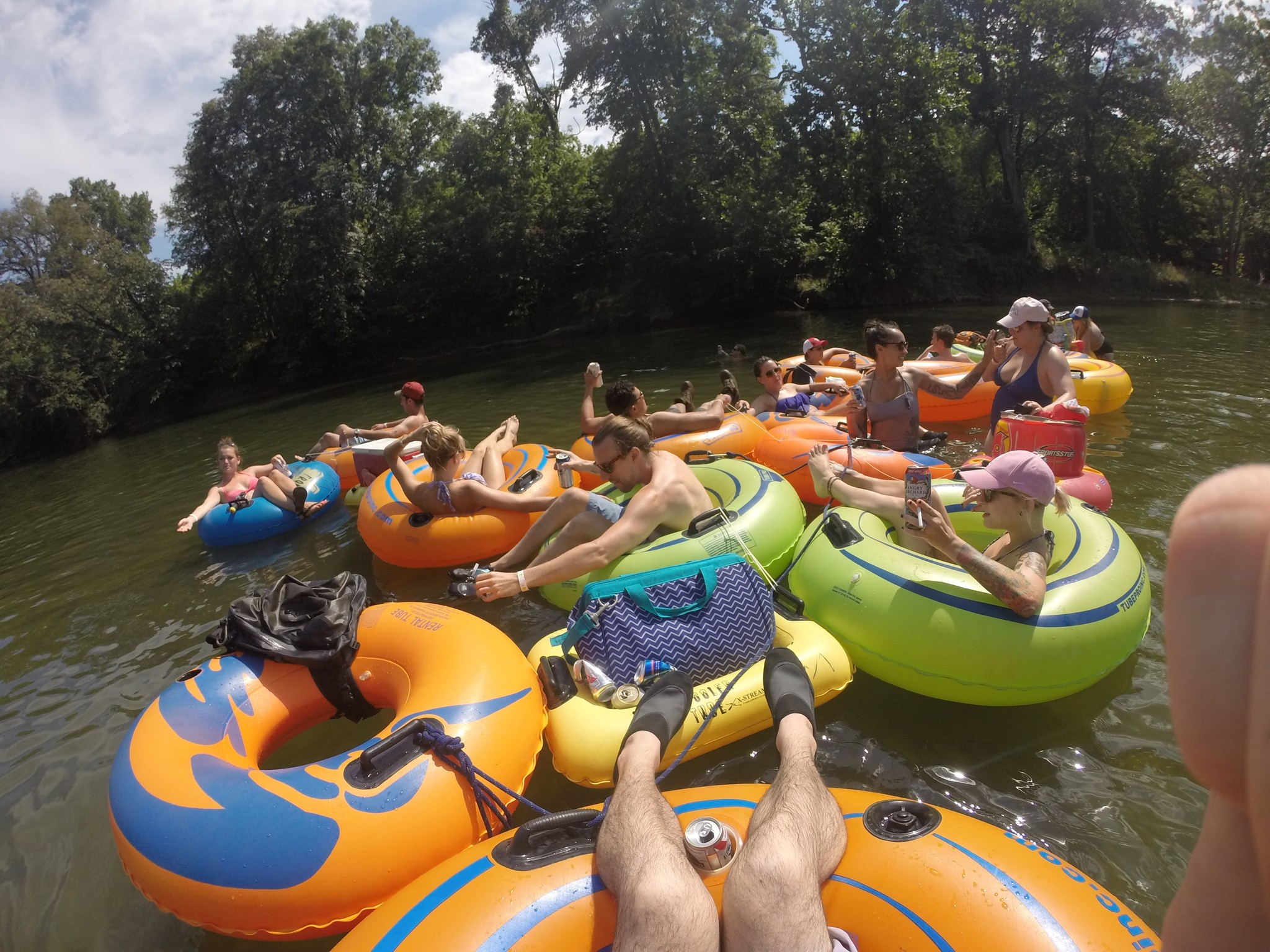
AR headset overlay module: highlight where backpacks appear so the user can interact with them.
[205,570,383,724]
[954,331,987,352]
[783,363,818,396]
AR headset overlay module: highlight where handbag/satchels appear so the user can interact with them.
[1048,317,1076,343]
[562,553,775,710]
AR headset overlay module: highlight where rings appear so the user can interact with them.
[976,489,978,493]
[970,486,974,491]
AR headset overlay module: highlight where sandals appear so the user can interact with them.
[298,500,330,523]
[292,487,308,514]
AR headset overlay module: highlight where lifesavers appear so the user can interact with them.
[1052,358,1134,415]
[787,477,1152,707]
[950,342,985,363]
[314,426,408,489]
[757,391,872,435]
[751,422,954,507]
[329,782,1167,952]
[777,353,876,387]
[570,412,768,491]
[537,457,808,612]
[1061,349,1094,359]
[901,360,1001,422]
[106,600,549,941]
[197,460,341,547]
[357,443,582,569]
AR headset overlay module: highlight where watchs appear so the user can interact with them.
[354,428,360,438]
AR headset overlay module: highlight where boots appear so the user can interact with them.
[720,370,740,405]
[675,380,695,412]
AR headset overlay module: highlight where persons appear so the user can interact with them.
[294,381,429,461]
[383,415,560,513]
[176,437,329,532]
[474,297,1114,644]
[594,648,847,952]
[1158,463,1270,952]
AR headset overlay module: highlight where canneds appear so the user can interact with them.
[589,362,603,388]
[849,384,867,409]
[684,816,734,871]
[556,453,574,489]
[904,464,932,531]
[848,353,856,362]
[611,683,646,709]
[634,659,678,688]
[573,658,617,703]
[718,345,723,354]
[273,459,292,477]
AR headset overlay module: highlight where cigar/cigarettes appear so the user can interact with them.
[916,504,923,528]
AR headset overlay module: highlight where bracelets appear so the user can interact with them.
[991,358,999,365]
[399,434,409,447]
[384,422,387,428]
[189,514,198,521]
[517,570,530,592]
[993,356,1000,363]
[712,399,723,405]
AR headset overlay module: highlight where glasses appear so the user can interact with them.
[766,367,782,378]
[1012,326,1021,333]
[218,441,235,447]
[631,391,645,404]
[812,346,825,351]
[882,341,908,351]
[594,449,632,474]
[983,489,1040,510]
[450,450,465,459]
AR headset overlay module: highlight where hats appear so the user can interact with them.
[959,450,1057,507]
[394,381,425,400]
[1038,298,1055,310]
[1070,306,1090,319]
[803,337,828,355]
[996,296,1049,329]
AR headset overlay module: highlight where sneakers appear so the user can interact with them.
[447,562,512,600]
[764,646,818,762]
[917,430,949,449]
[613,670,694,789]
